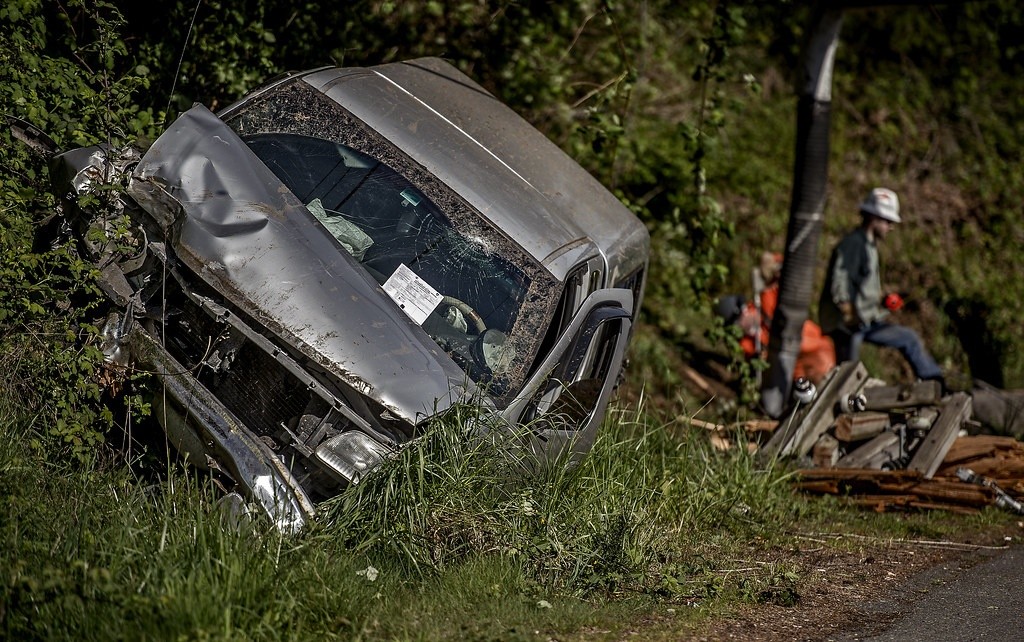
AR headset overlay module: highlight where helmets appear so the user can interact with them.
[861,188,902,224]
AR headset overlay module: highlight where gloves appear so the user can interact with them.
[839,300,867,334]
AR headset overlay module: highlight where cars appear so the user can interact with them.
[34,55,656,546]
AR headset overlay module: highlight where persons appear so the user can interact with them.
[737,188,950,397]
